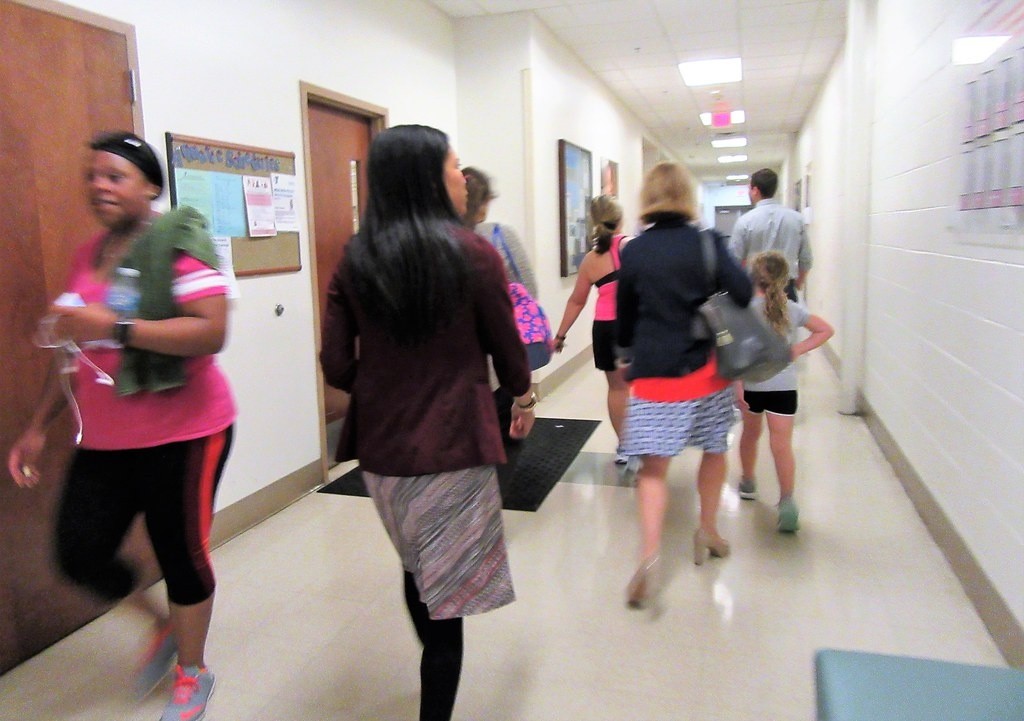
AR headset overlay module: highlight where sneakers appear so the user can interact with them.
[626,455,642,474]
[133,615,179,705]
[615,446,628,464]
[158,664,217,721]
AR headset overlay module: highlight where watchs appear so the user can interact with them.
[520,392,538,409]
[115,317,135,343]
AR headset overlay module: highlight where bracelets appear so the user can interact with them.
[557,335,565,340]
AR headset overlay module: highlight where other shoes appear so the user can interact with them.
[776,496,799,532]
[738,478,758,500]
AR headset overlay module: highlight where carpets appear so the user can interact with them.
[317,418,601,513]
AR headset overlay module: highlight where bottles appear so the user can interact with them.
[103,267,142,350]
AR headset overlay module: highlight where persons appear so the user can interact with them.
[551,194,636,464]
[735,250,834,533]
[613,162,753,609]
[457,166,538,491]
[8,131,240,721]
[727,168,814,303]
[319,124,535,721]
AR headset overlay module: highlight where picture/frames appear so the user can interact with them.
[601,157,619,197]
[560,139,594,276]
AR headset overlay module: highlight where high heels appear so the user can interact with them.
[625,550,665,604]
[693,529,731,565]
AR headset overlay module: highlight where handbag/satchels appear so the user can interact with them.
[492,224,551,372]
[696,227,791,384]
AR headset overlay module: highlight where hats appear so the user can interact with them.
[89,131,163,199]
